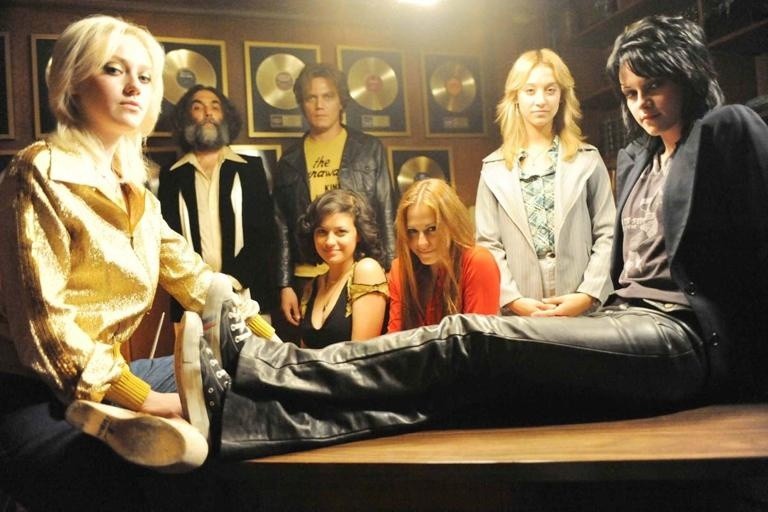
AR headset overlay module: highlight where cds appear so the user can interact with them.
[430,63,477,113]
[255,53,306,110]
[347,58,399,111]
[396,156,447,194]
[163,49,217,104]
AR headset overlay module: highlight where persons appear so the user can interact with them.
[159,85,280,323]
[386,178,501,333]
[273,63,395,328]
[297,189,391,350]
[175,16,768,461]
[0,14,283,512]
[475,48,617,315]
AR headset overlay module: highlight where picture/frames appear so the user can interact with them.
[417,49,485,139]
[0,35,19,142]
[335,41,415,142]
[242,39,323,141]
[227,141,284,164]
[143,145,184,169]
[0,147,22,174]
[386,145,459,200]
[29,32,60,141]
[145,34,231,140]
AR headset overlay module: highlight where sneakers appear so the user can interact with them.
[65,273,251,473]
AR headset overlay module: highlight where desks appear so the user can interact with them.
[209,404,768,511]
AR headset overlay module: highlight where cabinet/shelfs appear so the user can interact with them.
[511,0,767,172]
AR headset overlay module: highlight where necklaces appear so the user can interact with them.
[323,260,356,312]
